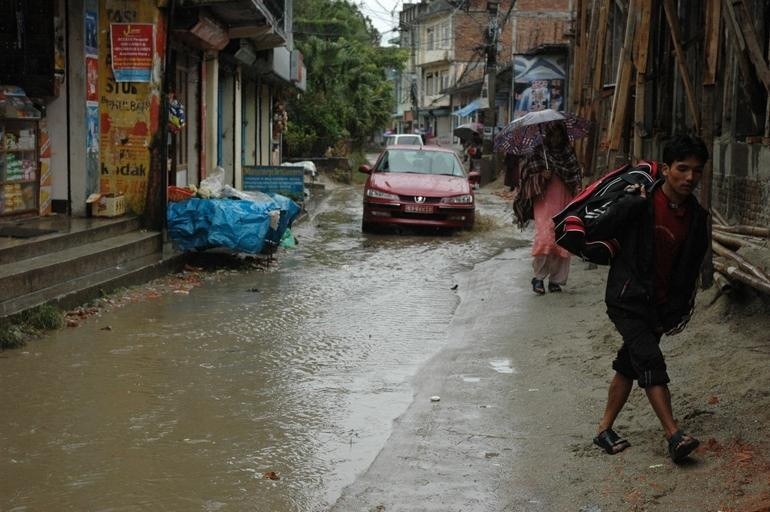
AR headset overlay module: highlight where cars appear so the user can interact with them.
[358,145,480,235]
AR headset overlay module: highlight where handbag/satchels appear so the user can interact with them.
[551,161,663,265]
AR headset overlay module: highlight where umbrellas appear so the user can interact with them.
[493,107,596,169]
[452,122,485,143]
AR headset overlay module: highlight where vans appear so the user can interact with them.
[383,133,425,145]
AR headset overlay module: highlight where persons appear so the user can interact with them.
[462,139,468,162]
[462,133,482,190]
[582,127,716,465]
[513,121,579,294]
[503,151,520,192]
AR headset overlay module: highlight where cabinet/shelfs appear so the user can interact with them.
[0,86,41,216]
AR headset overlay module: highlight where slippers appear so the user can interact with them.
[531,277,545,295]
[593,428,631,455]
[548,280,562,293]
[667,430,700,463]
